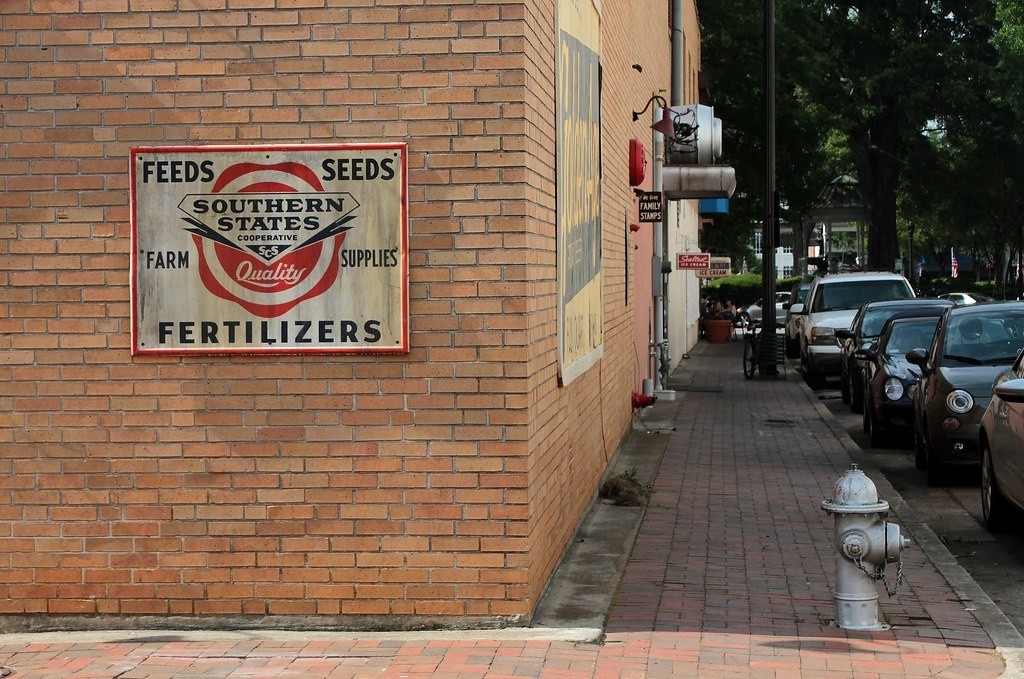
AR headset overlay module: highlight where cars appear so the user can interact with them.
[935,292,996,309]
[833,298,958,411]
[977,348,1024,534]
[905,300,1024,485]
[736,291,804,327]
[853,307,1019,449]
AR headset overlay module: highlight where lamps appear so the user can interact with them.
[632,95,676,139]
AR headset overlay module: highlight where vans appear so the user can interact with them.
[782,282,814,359]
[790,271,916,385]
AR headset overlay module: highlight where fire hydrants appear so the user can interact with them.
[820,462,912,633]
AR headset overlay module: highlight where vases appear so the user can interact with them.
[704,320,731,344]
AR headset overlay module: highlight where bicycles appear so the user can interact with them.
[742,320,763,379]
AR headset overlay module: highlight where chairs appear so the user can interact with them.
[898,329,926,348]
[954,317,994,358]
